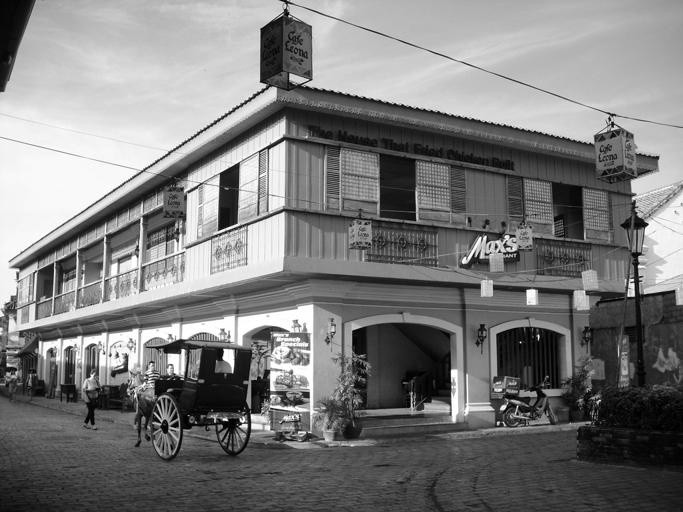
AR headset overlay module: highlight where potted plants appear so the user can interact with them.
[312,345,378,442]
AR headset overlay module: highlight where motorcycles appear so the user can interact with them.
[498,373,560,430]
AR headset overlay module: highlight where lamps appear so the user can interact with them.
[291,320,302,332]
[174,228,179,243]
[127,338,136,354]
[475,324,487,354]
[580,327,591,353]
[167,333,176,344]
[217,328,231,343]
[135,245,139,258]
[325,317,336,344]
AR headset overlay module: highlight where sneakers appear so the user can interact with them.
[83,424,96,429]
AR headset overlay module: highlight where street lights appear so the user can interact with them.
[621,198,649,390]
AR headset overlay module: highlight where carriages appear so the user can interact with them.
[123,332,254,461]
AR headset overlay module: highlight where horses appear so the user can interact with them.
[124,370,157,448]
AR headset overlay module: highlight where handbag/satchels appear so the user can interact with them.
[86,390,100,400]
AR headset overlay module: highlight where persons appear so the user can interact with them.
[159,364,177,380]
[4,367,19,402]
[142,360,160,386]
[215,349,233,373]
[23,368,39,401]
[80,369,101,429]
